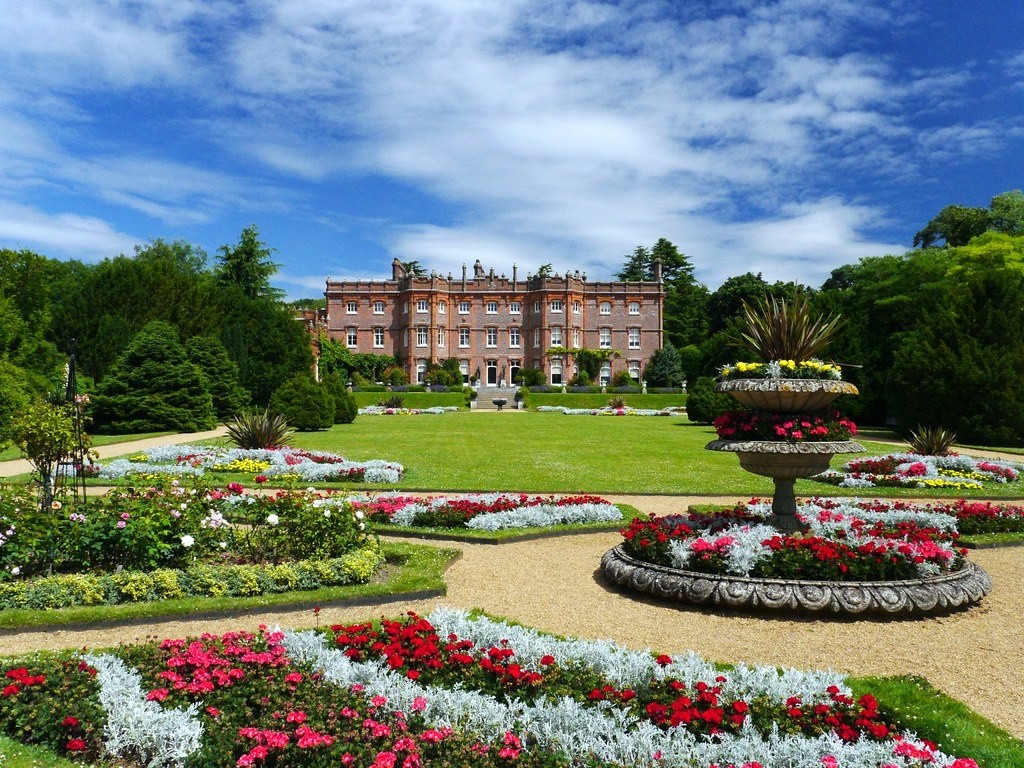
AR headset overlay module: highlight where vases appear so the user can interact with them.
[704,439,867,531]
[715,379,860,408]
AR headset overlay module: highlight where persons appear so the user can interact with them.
[502,362,506,378]
[476,366,480,379]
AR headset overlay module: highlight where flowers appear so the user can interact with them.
[716,407,860,444]
[717,359,845,380]
